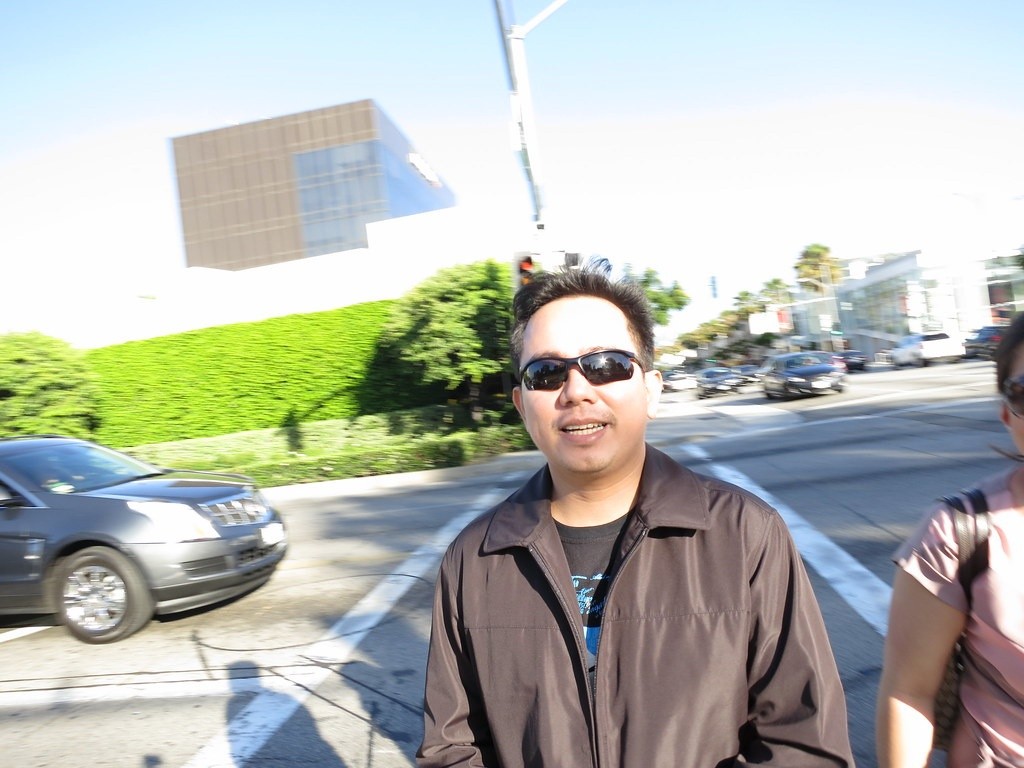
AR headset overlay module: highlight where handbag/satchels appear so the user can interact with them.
[927,476,992,755]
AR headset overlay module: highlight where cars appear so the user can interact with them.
[965,325,1010,358]
[1,437,286,645]
[891,331,966,368]
[663,349,868,402]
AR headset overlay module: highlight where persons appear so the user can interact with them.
[878,311,1024,768]
[415,274,855,768]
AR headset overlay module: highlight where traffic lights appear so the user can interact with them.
[517,254,535,287]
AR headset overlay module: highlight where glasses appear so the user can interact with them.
[519,345,648,393]
[996,373,1024,421]
